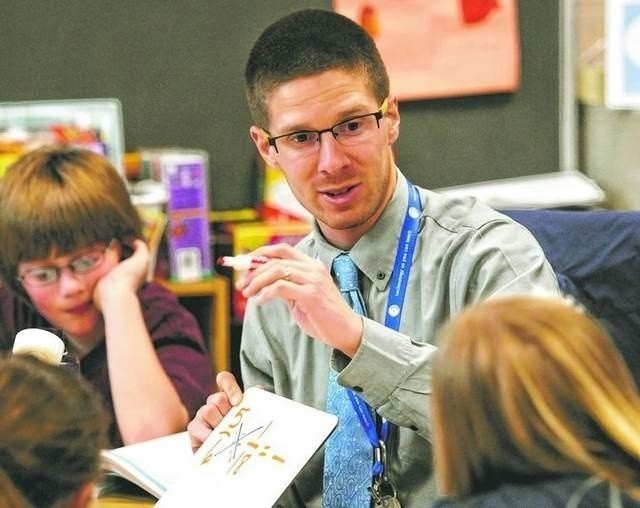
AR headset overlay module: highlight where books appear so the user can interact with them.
[90,430,195,501]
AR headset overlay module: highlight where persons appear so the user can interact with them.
[0,352,112,508]
[426,293,640,508]
[1,146,217,448]
[185,9,562,508]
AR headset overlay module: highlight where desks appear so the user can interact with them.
[147,270,232,378]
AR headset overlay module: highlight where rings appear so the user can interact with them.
[285,267,290,280]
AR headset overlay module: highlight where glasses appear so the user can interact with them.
[14,244,110,288]
[265,99,389,160]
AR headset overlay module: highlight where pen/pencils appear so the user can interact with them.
[217,254,270,272]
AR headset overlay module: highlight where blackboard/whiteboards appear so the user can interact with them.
[0,0,579,213]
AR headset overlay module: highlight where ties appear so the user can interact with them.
[321,256,373,508]
